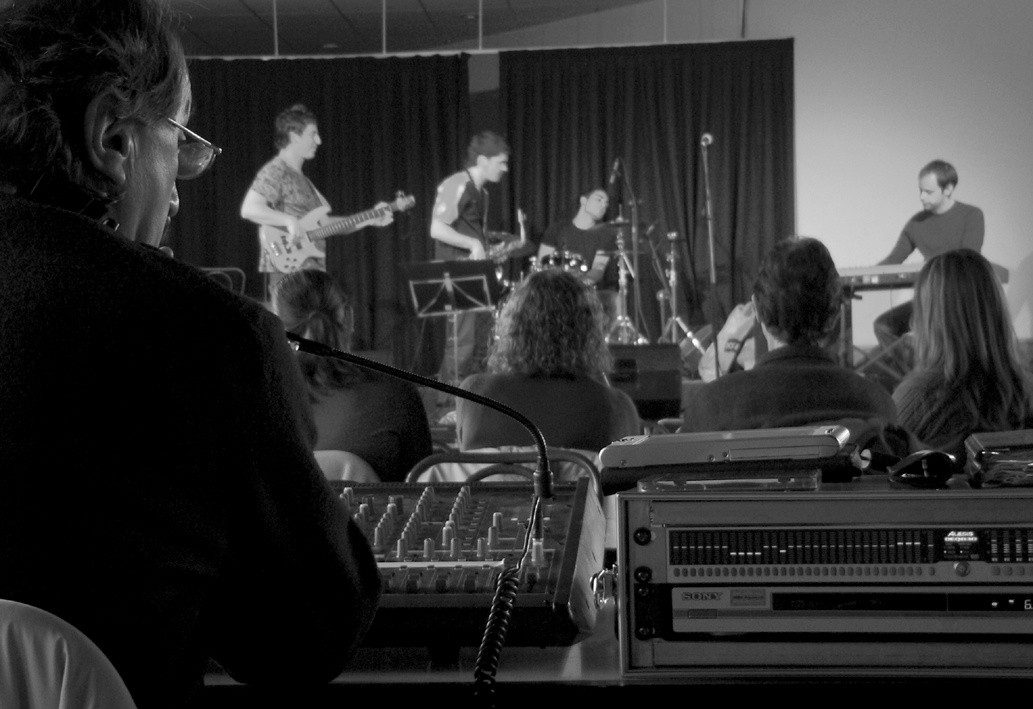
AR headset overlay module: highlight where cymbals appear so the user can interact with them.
[586,218,649,230]
[597,249,647,260]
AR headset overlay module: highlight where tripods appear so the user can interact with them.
[604,166,705,356]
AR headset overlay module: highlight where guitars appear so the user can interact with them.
[259,192,419,277]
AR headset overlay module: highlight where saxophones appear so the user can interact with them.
[461,208,529,283]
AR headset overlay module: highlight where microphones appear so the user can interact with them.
[609,159,618,183]
[284,331,554,500]
[701,133,713,146]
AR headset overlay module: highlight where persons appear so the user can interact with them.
[0,0,385,709]
[535,184,616,286]
[273,269,434,481]
[683,236,898,432]
[456,271,642,453]
[238,104,393,319]
[873,161,1032,448]
[430,132,511,424]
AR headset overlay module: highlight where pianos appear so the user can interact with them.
[839,261,1011,376]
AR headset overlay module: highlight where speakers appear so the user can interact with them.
[606,344,683,421]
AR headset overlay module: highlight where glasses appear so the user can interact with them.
[158,113,222,180]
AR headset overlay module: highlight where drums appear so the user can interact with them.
[540,251,590,279]
